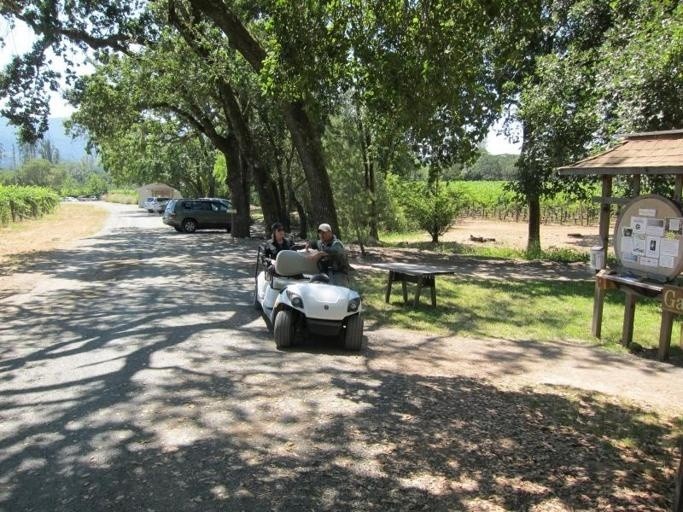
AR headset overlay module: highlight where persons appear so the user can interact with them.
[307,222,349,287]
[262,221,303,279]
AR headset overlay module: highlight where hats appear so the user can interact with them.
[271,223,282,230]
[318,224,331,232]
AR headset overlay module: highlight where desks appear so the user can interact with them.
[370,262,455,310]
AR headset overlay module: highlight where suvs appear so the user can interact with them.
[144,197,171,213]
[162,199,252,234]
[251,239,363,352]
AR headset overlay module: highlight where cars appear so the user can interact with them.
[157,202,168,213]
